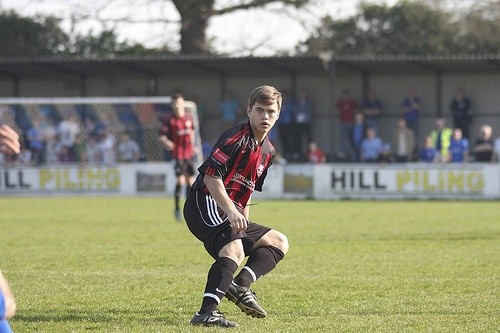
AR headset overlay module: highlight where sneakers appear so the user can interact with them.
[190,308,239,329]
[224,278,267,319]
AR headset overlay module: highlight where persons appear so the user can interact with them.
[0,268,16,333]
[0,114,141,169]
[219,77,497,162]
[160,92,200,222]
[183,85,289,328]
